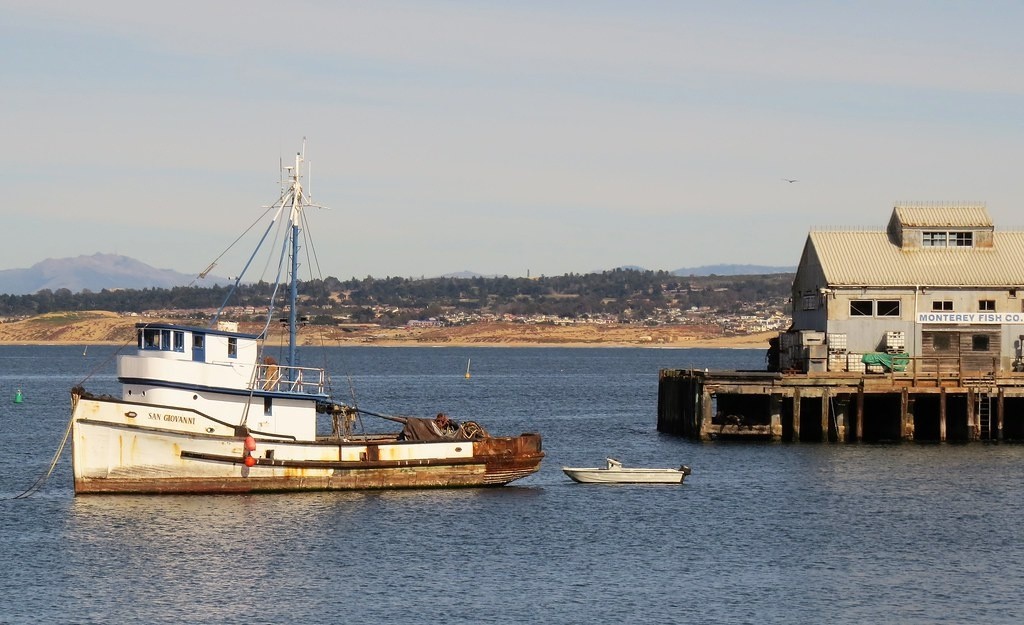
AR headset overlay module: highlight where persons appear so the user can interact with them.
[262,354,278,391]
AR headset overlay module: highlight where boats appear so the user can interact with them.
[561,456,690,485]
[71,136,546,497]
